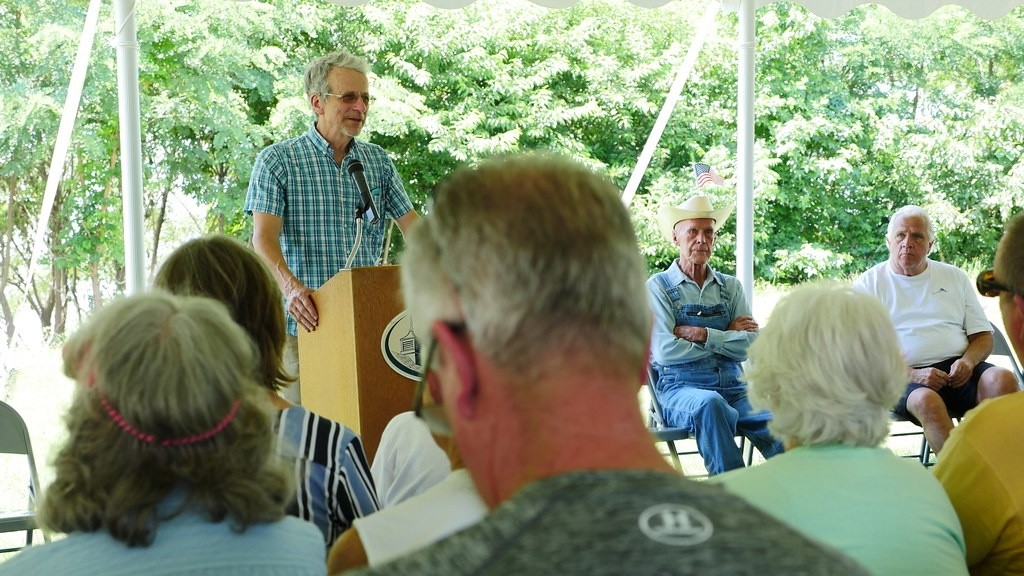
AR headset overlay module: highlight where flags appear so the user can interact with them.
[695,163,725,189]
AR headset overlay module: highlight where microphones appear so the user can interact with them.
[347,159,380,224]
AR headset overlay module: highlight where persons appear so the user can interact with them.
[329,146,1023,576]
[241,52,423,406]
[0,224,380,576]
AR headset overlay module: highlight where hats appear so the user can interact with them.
[658,195,731,244]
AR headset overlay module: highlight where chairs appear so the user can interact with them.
[0,400,52,554]
[643,361,772,479]
[891,322,1024,469]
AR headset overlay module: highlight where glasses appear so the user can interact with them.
[415,318,464,436]
[976,268,1024,298]
[316,92,377,106]
